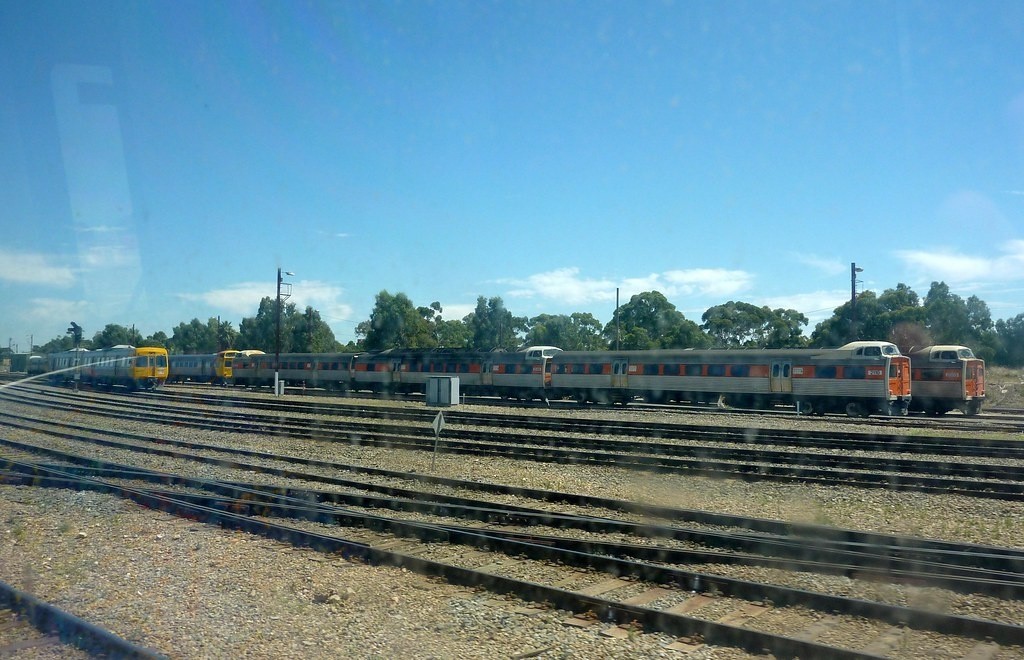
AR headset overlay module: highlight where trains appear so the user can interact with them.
[27,347,169,391]
[231,340,985,418]
[168,350,239,386]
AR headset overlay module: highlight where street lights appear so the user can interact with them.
[852,262,864,304]
[276,268,295,371]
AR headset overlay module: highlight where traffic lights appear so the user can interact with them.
[68,322,82,344]
